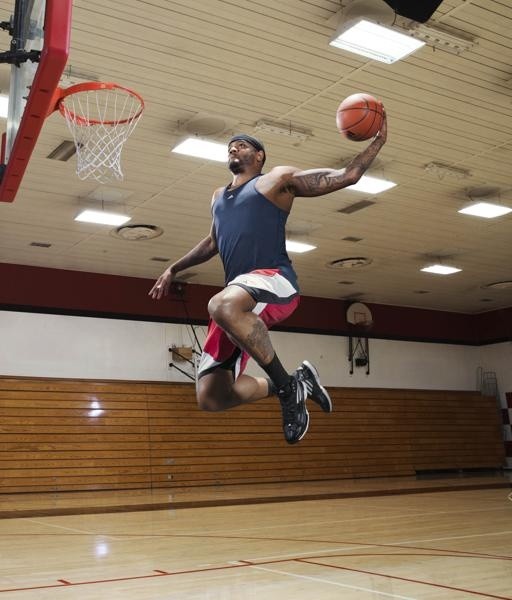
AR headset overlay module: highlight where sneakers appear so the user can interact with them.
[295,360,333,413]
[272,375,310,445]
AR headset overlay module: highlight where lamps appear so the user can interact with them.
[419,257,465,278]
[326,19,428,68]
[75,207,134,231]
[284,237,315,256]
[345,170,399,196]
[166,136,232,167]
[451,189,511,225]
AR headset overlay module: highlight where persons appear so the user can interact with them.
[149,104,387,443]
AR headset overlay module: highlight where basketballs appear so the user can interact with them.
[336,92,385,141]
